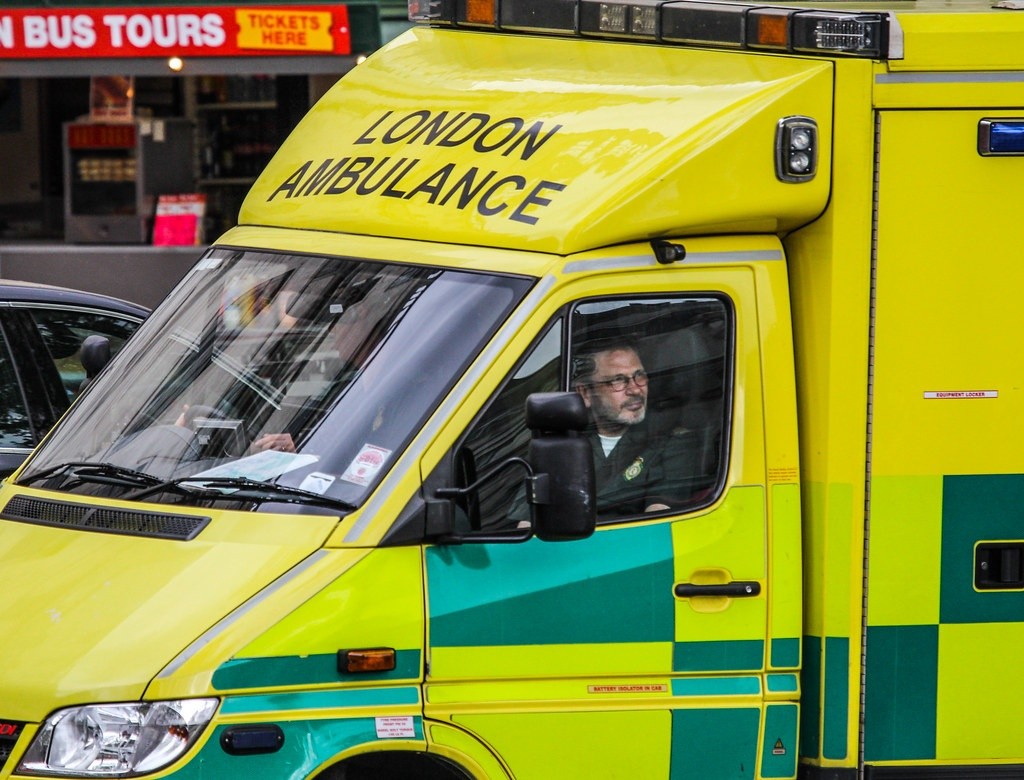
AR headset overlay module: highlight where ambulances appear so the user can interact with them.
[0,0,1024,780]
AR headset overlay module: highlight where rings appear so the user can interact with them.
[282,447,285,451]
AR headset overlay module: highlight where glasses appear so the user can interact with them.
[590,370,650,392]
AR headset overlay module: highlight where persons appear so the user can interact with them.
[172,302,390,458]
[515,331,701,530]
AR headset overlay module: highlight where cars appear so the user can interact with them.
[0,279,292,489]
